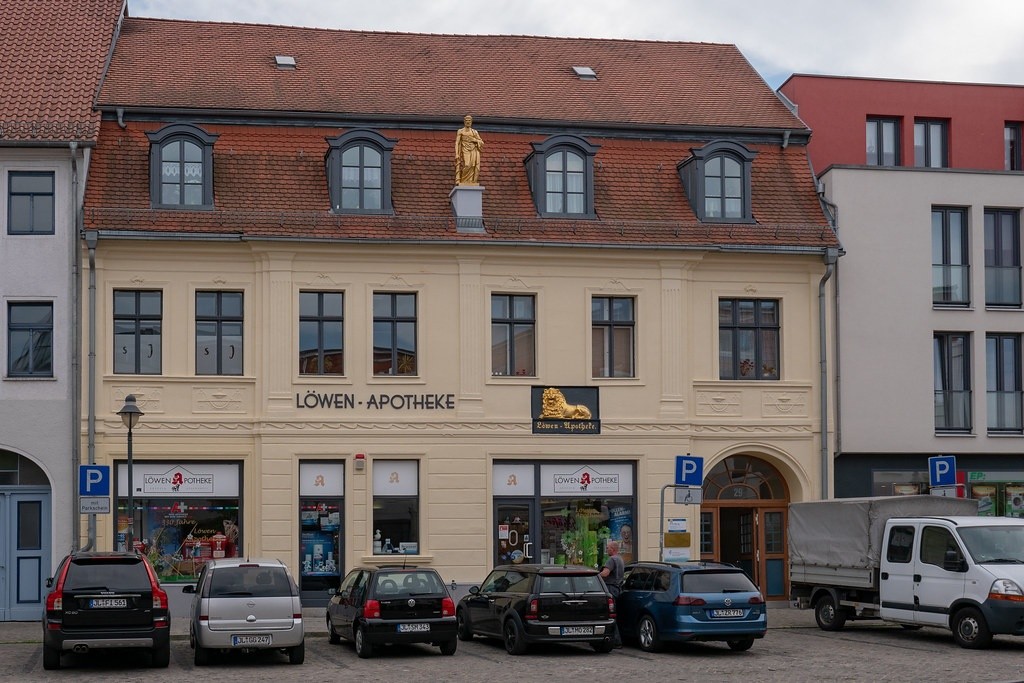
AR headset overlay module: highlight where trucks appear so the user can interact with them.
[788,494,1024,650]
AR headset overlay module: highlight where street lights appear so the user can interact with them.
[116,395,145,553]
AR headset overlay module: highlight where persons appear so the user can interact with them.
[599,541,624,649]
[619,524,632,553]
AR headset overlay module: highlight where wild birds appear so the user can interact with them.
[373,529,382,541]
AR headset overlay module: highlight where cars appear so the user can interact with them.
[326,565,459,659]
[456,565,616,656]
[181,559,306,666]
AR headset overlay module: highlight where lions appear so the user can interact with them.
[539,388,592,420]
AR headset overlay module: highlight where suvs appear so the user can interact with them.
[42,553,171,671]
[618,560,768,653]
